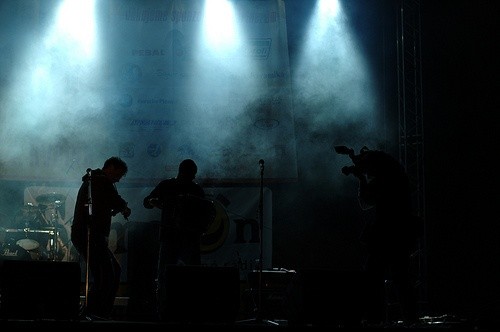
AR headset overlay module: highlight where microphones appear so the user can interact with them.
[258,159,264,176]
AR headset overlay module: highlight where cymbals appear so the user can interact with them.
[20,205,38,213]
[35,193,65,203]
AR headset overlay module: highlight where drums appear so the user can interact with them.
[15,233,40,250]
[198,198,230,254]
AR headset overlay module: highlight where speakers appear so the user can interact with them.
[0,258,81,323]
[156,264,240,323]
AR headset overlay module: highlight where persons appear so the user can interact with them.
[70,156,132,320]
[144,157,215,324]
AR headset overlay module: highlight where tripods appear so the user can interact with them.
[236,173,280,326]
[80,167,108,321]
